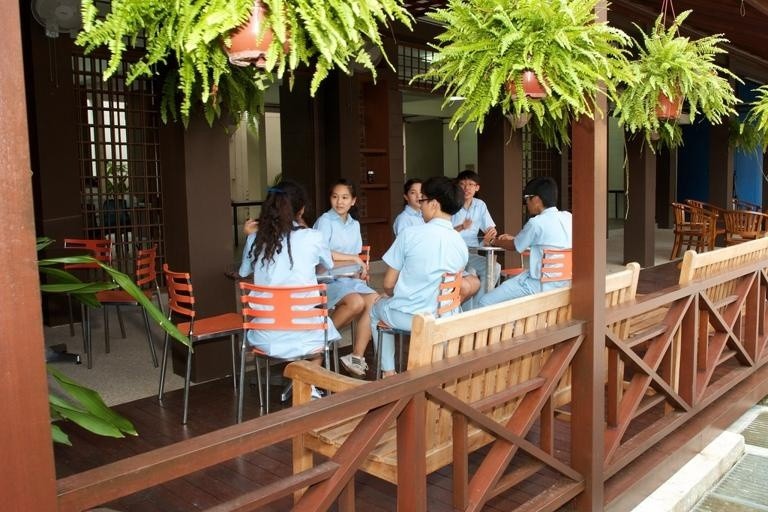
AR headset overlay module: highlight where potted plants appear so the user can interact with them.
[72,0,418,139]
[607,0,746,156]
[410,0,640,152]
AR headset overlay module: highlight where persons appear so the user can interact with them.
[391,178,480,305]
[313,179,380,379]
[368,174,468,380]
[452,168,501,311]
[469,175,572,308]
[236,176,332,403]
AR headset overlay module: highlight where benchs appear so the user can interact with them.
[628,237,768,414]
[281,263,639,509]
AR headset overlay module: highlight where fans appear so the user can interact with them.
[31,0,83,38]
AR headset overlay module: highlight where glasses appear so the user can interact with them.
[419,199,435,204]
[522,194,536,200]
[460,183,479,188]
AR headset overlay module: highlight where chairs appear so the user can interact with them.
[336,238,573,294]
[376,266,464,387]
[61,238,126,352]
[84,198,137,253]
[157,262,263,423]
[237,278,340,424]
[667,195,768,263]
[86,245,167,370]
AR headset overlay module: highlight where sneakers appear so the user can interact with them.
[312,386,323,399]
[339,353,369,379]
